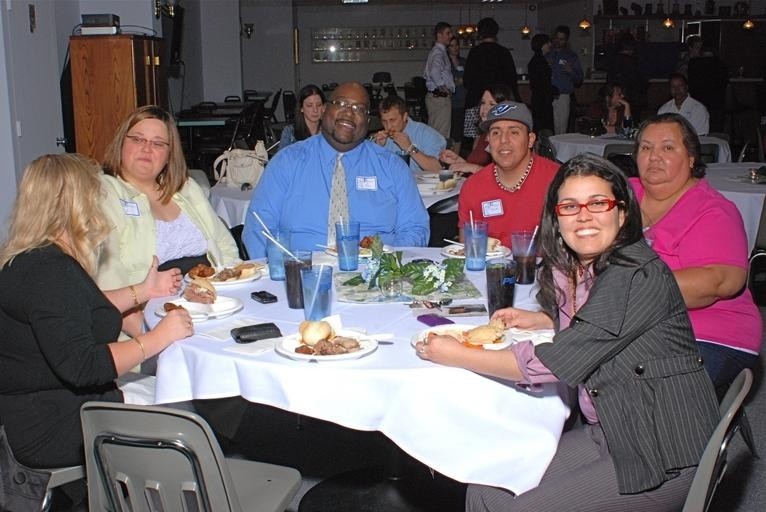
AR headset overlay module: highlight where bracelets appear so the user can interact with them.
[128,285,138,306]
[134,336,146,364]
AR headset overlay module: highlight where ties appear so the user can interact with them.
[328,153,349,251]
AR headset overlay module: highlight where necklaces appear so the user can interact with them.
[493,156,534,192]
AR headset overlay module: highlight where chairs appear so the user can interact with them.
[224,96,241,103]
[262,88,283,144]
[710,132,730,142]
[603,143,639,177]
[699,143,719,163]
[283,90,296,122]
[0,424,86,512]
[199,102,216,106]
[220,102,259,151]
[738,140,752,162]
[80,401,302,512]
[322,72,428,126]
[538,128,552,156]
[245,90,257,95]
[681,366,753,512]
[715,249,766,460]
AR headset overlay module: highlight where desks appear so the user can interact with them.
[248,92,272,101]
[212,106,247,149]
[175,117,231,169]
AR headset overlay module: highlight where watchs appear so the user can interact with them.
[409,145,419,155]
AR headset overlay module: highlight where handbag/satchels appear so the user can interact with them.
[214,141,269,190]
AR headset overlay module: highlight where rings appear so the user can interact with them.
[423,337,428,344]
[419,346,426,354]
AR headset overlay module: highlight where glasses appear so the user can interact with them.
[555,200,621,217]
[125,134,171,151]
[327,100,369,115]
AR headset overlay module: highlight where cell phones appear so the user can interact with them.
[251,291,277,304]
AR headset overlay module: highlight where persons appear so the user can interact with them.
[439,87,511,174]
[1,156,194,471]
[412,152,721,512]
[460,17,518,155]
[240,81,431,264]
[423,21,455,140]
[90,104,240,372]
[546,25,584,135]
[679,35,706,73]
[527,34,560,141]
[276,84,326,151]
[634,111,763,404]
[606,33,655,111]
[447,36,466,143]
[455,100,563,257]
[657,73,710,138]
[578,82,632,135]
[369,94,448,174]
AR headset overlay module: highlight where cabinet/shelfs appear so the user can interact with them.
[308,24,480,64]
[69,35,168,173]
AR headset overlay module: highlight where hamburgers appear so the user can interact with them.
[488,238,501,251]
[470,325,503,344]
[304,321,331,343]
[438,179,456,189]
[184,280,215,304]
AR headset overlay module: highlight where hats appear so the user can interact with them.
[479,102,534,133]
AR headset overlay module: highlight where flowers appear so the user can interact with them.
[341,252,466,296]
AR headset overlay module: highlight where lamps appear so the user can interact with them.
[521,5,531,35]
[578,0,591,29]
[663,0,673,27]
[465,8,474,33]
[743,15,754,29]
[155,0,179,19]
[456,7,465,35]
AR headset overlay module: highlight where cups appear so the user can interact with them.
[464,221,487,270]
[484,259,518,322]
[621,116,634,139]
[299,266,334,324]
[337,221,361,270]
[266,231,290,281]
[512,231,538,284]
[284,249,312,308]
[439,170,453,182]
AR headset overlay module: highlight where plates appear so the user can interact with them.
[184,268,260,287]
[325,244,395,255]
[276,330,377,362]
[412,325,511,350]
[154,296,244,321]
[440,244,512,258]
[418,172,463,184]
